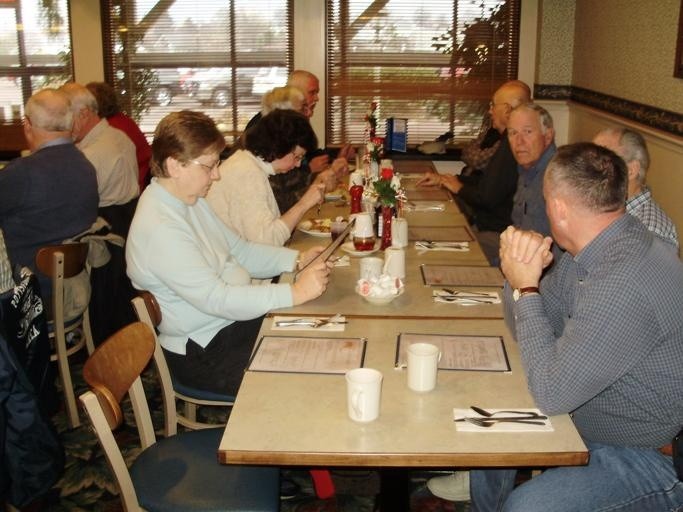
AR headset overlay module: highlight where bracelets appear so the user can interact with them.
[296,250,304,273]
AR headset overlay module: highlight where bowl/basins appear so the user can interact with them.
[355,284,405,304]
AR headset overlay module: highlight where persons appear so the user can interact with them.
[86,83,152,194]
[200,109,324,246]
[57,84,141,317]
[0,88,100,357]
[487,102,564,281]
[125,111,336,503]
[426,142,683,512]
[416,80,531,258]
[592,127,679,259]
[263,86,348,217]
[245,70,355,163]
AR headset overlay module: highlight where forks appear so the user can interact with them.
[436,293,493,304]
[464,416,545,427]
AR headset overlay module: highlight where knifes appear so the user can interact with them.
[431,295,497,299]
[282,320,349,325]
[454,415,547,422]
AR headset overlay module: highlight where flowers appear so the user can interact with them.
[364,102,384,160]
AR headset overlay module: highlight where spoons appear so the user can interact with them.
[470,406,538,417]
[442,288,489,295]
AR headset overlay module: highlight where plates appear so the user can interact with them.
[339,241,380,256]
[324,196,343,201]
[297,219,332,237]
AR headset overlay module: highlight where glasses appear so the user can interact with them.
[291,151,303,161]
[489,102,504,106]
[189,159,220,171]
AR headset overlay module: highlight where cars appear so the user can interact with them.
[253,66,288,98]
[189,67,254,105]
[143,69,184,106]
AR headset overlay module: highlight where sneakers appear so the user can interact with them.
[427,470,471,501]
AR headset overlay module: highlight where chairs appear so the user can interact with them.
[131,291,234,438]
[79,321,280,512]
[35,243,95,428]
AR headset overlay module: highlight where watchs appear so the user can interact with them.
[512,287,539,302]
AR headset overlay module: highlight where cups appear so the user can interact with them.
[344,367,383,423]
[330,222,347,245]
[391,218,409,248]
[405,343,442,393]
[383,246,406,279]
[359,257,384,279]
[352,212,377,250]
[348,172,363,191]
